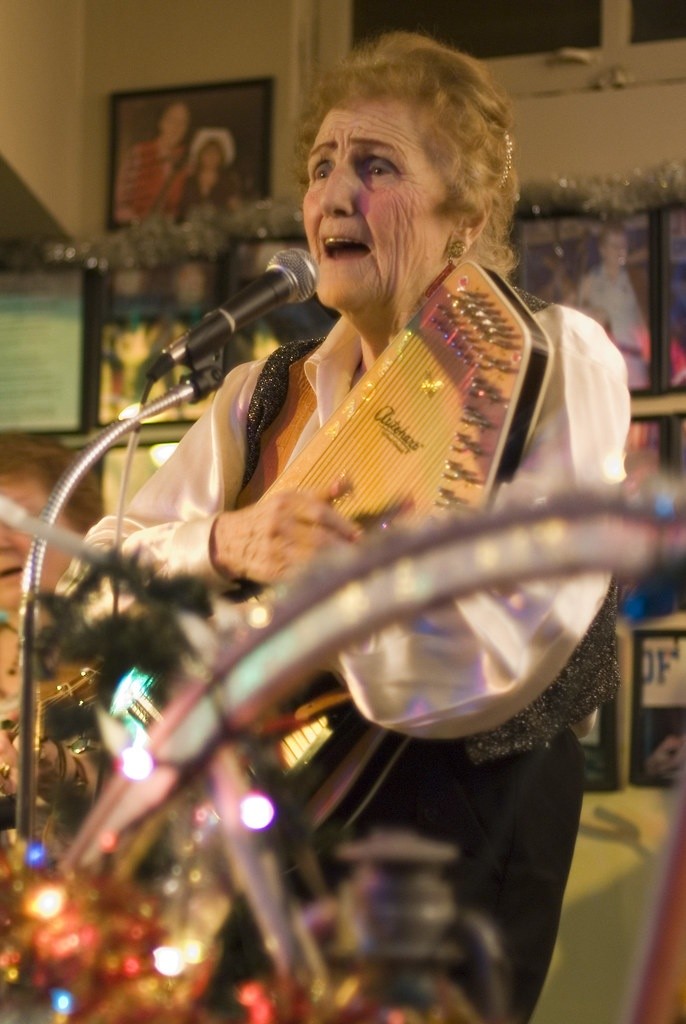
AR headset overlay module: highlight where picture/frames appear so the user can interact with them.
[628,629,686,788]
[657,204,686,395]
[0,238,343,439]
[509,207,660,396]
[104,76,272,230]
[569,697,621,792]
[624,416,671,479]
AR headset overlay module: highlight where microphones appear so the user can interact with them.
[145,248,320,381]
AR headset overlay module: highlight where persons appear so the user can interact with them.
[111,97,242,225]
[577,223,648,360]
[53,36,631,1024]
[0,429,108,830]
